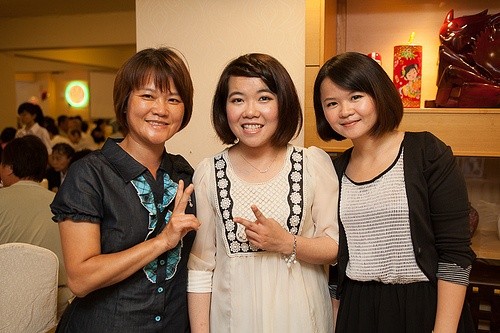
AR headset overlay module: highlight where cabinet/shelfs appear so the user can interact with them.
[305,0,500,287]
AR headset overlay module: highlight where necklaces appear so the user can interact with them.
[237,142,281,173]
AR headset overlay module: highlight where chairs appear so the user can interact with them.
[0,243,62,333]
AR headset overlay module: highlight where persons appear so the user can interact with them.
[48,47,195,333]
[186,52,341,333]
[0,100,127,192]
[313,51,479,333]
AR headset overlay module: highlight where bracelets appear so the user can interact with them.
[280,233,297,265]
